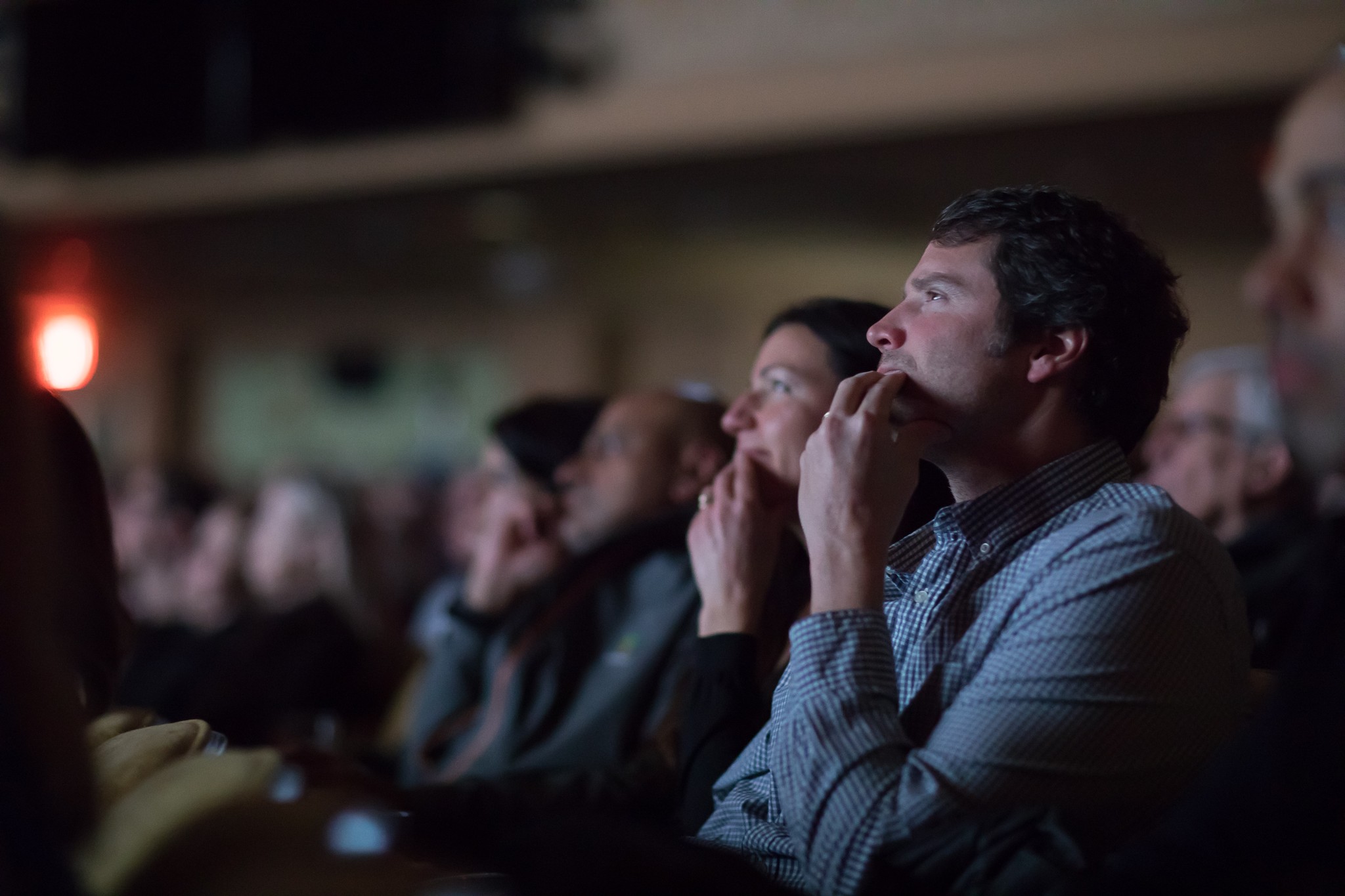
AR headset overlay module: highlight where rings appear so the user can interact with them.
[821,411,845,420]
[698,494,712,508]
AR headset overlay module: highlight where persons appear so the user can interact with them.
[1,73,1345,896]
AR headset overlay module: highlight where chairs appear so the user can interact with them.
[78,707,298,896]
